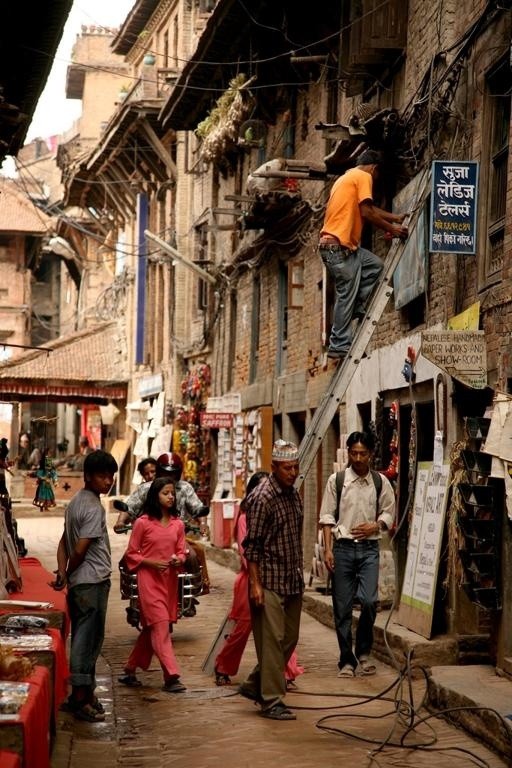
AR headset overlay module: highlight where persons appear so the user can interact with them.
[136,456,157,482]
[212,470,305,691]
[44,446,118,722]
[235,439,306,723]
[67,437,96,471]
[319,430,396,680]
[34,447,59,512]
[114,452,207,625]
[1,438,19,500]
[310,146,413,358]
[118,476,192,693]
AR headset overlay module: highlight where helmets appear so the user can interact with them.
[156,452,183,478]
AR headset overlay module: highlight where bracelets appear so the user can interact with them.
[376,522,383,533]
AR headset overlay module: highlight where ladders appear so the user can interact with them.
[202,169,432,677]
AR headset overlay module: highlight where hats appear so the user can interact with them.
[271,439,300,461]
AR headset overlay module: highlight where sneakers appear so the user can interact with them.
[327,352,367,359]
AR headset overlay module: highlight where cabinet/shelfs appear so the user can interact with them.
[0,556,71,768]
[210,406,273,551]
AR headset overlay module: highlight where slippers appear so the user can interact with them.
[237,687,297,720]
[359,655,377,675]
[162,682,187,691]
[117,675,143,687]
[338,664,355,678]
[60,694,104,722]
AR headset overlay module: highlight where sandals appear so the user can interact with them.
[285,680,297,690]
[213,666,231,686]
